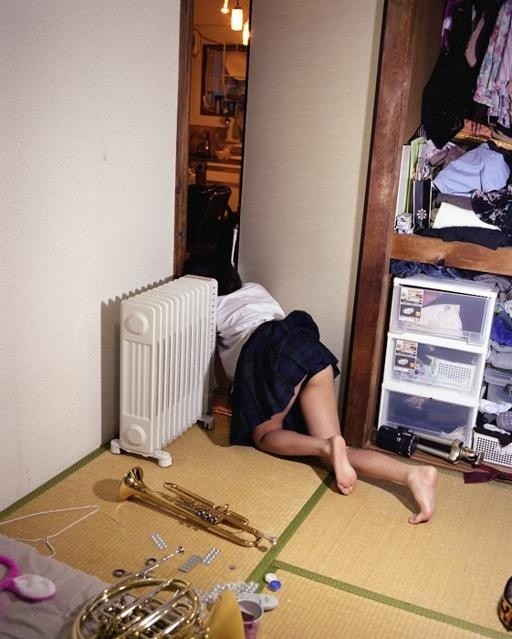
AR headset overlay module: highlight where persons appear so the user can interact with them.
[183,245,439,524]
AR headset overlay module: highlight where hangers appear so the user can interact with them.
[1,504,101,559]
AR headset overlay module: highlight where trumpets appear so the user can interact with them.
[118,468,278,547]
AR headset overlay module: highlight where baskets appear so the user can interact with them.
[435,359,476,392]
[472,430,512,468]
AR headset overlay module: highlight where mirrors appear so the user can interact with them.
[200,43,247,117]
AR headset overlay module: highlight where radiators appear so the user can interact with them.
[108,274,224,467]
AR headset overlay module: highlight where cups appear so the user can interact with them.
[238,598,264,638]
[376,422,418,460]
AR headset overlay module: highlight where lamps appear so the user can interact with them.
[230,0,245,32]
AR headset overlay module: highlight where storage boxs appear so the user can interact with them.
[376,275,512,450]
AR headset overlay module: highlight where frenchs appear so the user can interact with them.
[71,546,245,638]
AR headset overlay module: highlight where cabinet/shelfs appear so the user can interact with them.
[342,1,511,484]
